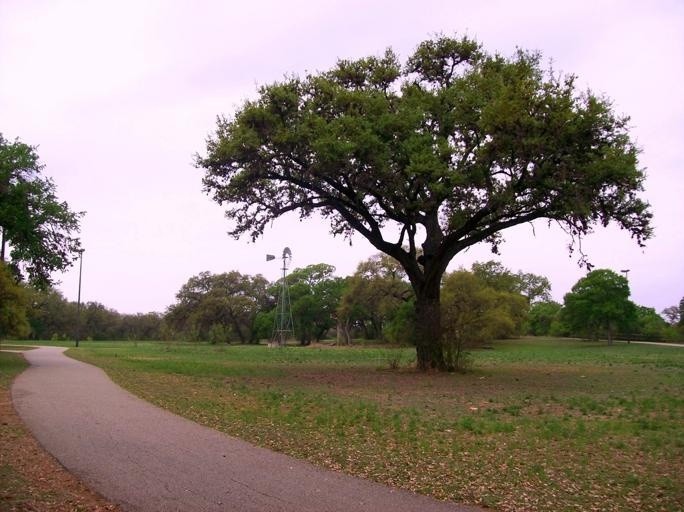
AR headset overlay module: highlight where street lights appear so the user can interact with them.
[73,249,85,347]
